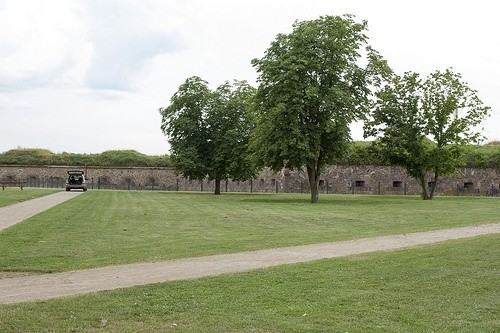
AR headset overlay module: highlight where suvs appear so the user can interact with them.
[66,170,87,192]
